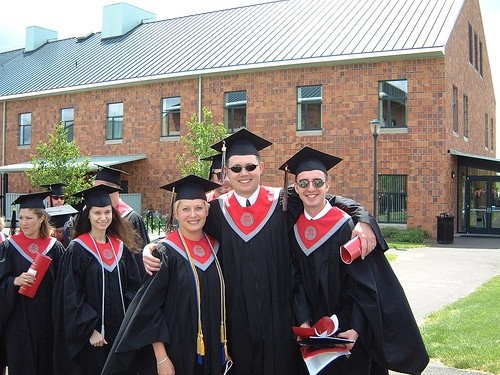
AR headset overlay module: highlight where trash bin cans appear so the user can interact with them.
[436,213,455,244]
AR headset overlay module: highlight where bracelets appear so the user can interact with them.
[157,356,169,364]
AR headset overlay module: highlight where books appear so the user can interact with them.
[292,314,355,375]
[18,254,52,297]
[340,236,362,264]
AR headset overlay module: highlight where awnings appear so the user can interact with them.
[0,155,146,177]
[452,153,500,172]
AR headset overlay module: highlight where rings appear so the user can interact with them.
[371,244,375,245]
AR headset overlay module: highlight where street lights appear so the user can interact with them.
[369,117,382,221]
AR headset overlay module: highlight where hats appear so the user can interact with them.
[210,128,273,168]
[71,185,123,207]
[160,174,221,234]
[41,183,69,195]
[279,146,343,211]
[95,164,130,186]
[11,192,52,236]
[199,154,223,180]
[45,205,79,229]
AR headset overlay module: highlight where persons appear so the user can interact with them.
[0,192,65,375]
[278,146,430,375]
[40,183,69,207]
[200,153,232,202]
[94,162,149,250]
[101,175,232,375]
[53,184,142,375]
[142,126,390,375]
[0,217,5,243]
[46,205,79,250]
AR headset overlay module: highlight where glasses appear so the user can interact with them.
[52,196,64,200]
[298,179,326,188]
[214,172,226,179]
[228,164,258,173]
